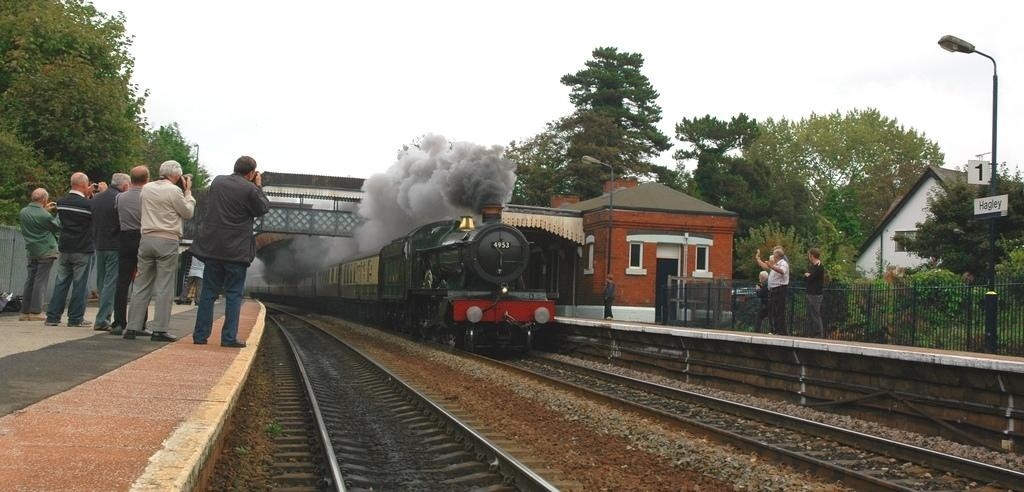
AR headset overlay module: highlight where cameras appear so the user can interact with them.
[251,171,260,184]
[176,173,194,190]
[52,202,56,212]
[93,185,99,192]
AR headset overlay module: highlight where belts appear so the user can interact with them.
[767,284,787,292]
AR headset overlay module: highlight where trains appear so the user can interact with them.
[246,204,557,353]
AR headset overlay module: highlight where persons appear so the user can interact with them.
[89,173,130,330]
[603,274,615,320]
[769,246,790,264]
[185,156,270,348]
[109,164,155,337]
[754,270,768,332]
[803,247,824,337]
[752,246,789,336]
[43,172,108,328]
[19,187,60,320]
[123,160,194,343]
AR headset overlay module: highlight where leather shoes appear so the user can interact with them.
[110,326,122,335]
[221,340,247,348]
[124,330,136,339]
[193,339,208,344]
[135,328,153,336]
[150,331,176,342]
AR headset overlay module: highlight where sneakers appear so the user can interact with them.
[768,330,787,337]
[68,319,93,327]
[29,313,45,321]
[19,313,30,321]
[94,323,112,331]
[45,320,57,327]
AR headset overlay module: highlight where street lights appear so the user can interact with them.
[937,32,998,350]
[191,142,200,181]
[581,155,614,318]
